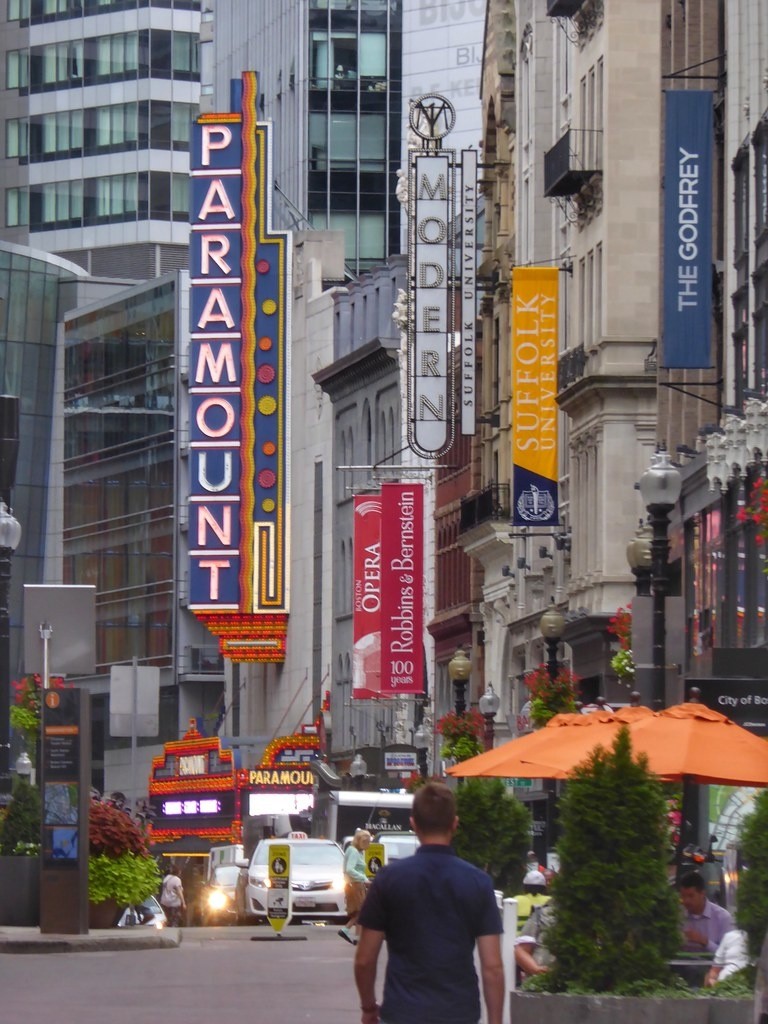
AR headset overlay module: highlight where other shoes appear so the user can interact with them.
[338,927,355,943]
[353,936,360,944]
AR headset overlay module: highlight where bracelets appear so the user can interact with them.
[361,1004,378,1011]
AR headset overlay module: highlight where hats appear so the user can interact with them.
[527,851,535,856]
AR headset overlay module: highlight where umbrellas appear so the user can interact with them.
[446,686,768,888]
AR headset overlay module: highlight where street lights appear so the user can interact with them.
[639,434,681,714]
[478,680,500,755]
[627,516,654,597]
[414,724,431,781]
[539,600,565,682]
[449,642,473,792]
[0,499,21,806]
[349,753,368,791]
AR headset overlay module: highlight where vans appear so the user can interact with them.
[515,789,564,840]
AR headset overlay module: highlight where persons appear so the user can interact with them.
[513,906,557,978]
[706,930,758,988]
[680,869,733,950]
[354,782,506,1024]
[337,830,371,945]
[514,871,551,935]
[161,865,186,927]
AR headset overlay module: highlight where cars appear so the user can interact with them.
[197,862,247,926]
[233,831,350,926]
[344,830,422,881]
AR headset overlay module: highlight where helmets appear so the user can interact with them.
[523,871,546,886]
